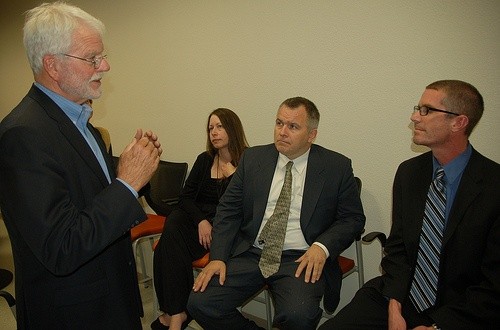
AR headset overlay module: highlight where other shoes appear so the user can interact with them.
[150,313,190,330]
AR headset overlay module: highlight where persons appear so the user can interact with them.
[150,106,254,330]
[0,0,163,330]
[189,96,366,330]
[318,80,500,330]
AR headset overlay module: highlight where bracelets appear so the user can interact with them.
[432,323,440,330]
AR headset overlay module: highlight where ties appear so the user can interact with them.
[410,168,447,313]
[259,161,292,279]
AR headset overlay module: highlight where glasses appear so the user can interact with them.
[414,105,459,117]
[62,54,107,68]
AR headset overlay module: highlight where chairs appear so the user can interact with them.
[239,176,365,330]
[130,160,188,288]
